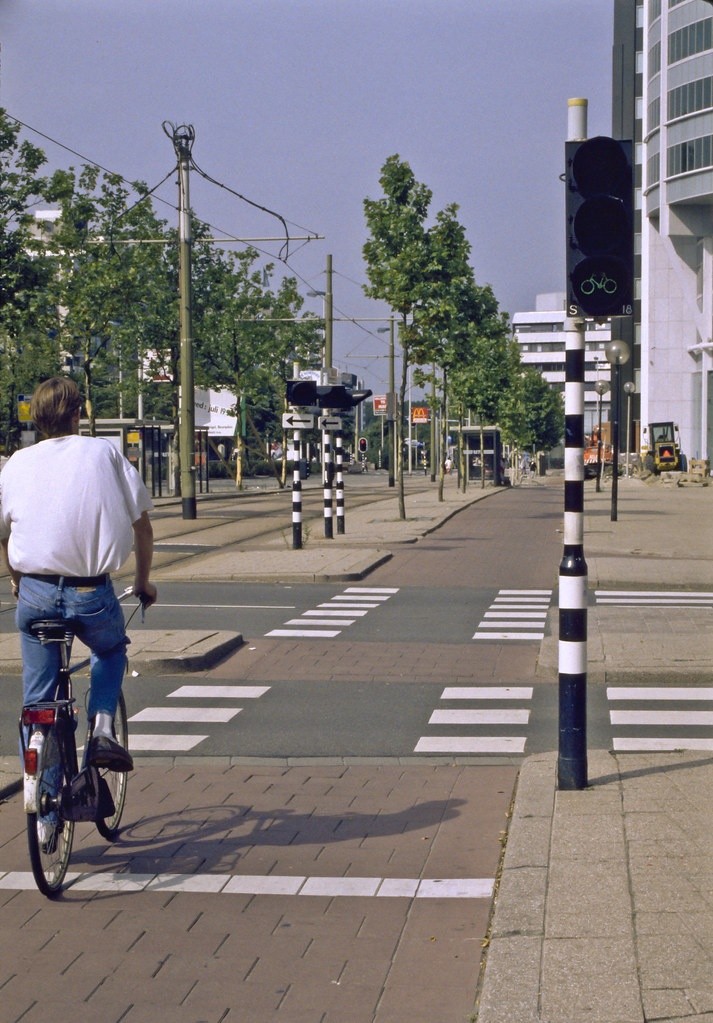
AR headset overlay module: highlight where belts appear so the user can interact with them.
[24,572,107,587]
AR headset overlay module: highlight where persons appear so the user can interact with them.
[529,461,536,479]
[445,458,452,475]
[0,375,158,854]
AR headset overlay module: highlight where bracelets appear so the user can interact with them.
[10,580,19,587]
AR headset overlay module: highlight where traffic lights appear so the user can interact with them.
[562,135,636,315]
[283,379,374,411]
[357,438,367,453]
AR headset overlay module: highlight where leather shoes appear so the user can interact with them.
[42,820,64,854]
[88,736,133,772]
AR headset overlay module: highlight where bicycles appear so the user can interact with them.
[18,585,148,898]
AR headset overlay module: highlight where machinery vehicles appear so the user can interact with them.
[584,423,615,481]
[642,422,688,476]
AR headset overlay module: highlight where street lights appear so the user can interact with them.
[624,382,635,478]
[307,289,333,373]
[376,314,396,487]
[604,340,631,522]
[594,379,609,492]
